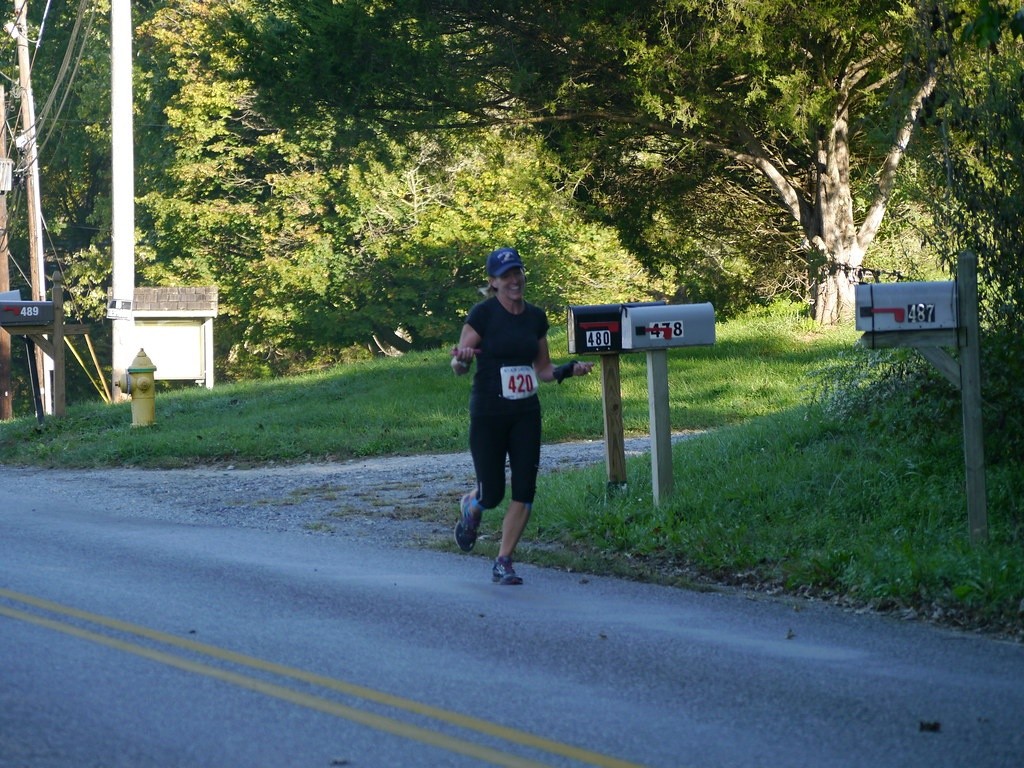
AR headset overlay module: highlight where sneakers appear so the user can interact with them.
[492,556,523,585]
[454,495,482,552]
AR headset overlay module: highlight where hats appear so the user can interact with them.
[487,247,524,276]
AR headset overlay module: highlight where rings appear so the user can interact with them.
[583,369,587,374]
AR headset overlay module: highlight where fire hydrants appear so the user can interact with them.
[115,349,157,428]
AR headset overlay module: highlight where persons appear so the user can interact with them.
[450,247,595,585]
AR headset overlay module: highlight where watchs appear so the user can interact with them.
[457,360,471,369]
